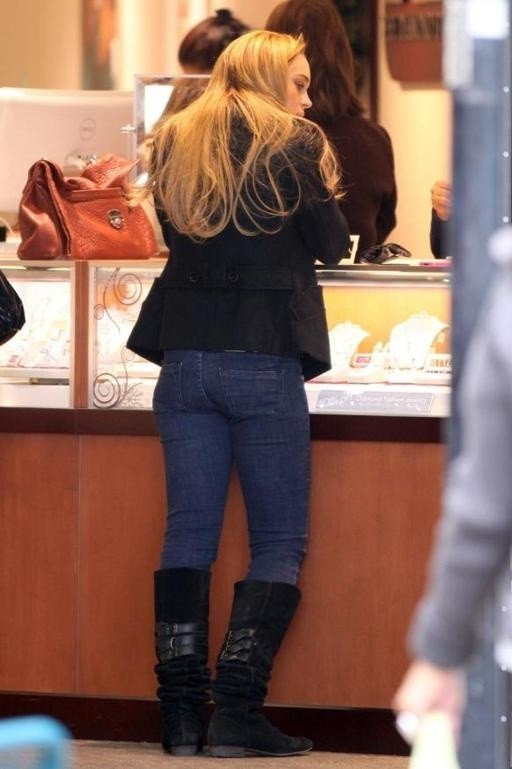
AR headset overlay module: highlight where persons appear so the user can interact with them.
[148,8,252,137]
[124,27,352,759]
[428,178,453,260]
[264,0,399,265]
[390,221,512,769]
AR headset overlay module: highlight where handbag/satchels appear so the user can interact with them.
[15,153,162,271]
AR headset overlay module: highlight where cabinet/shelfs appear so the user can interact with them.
[1,258,454,755]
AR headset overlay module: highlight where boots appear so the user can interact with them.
[202,571,317,762]
[149,568,216,761]
